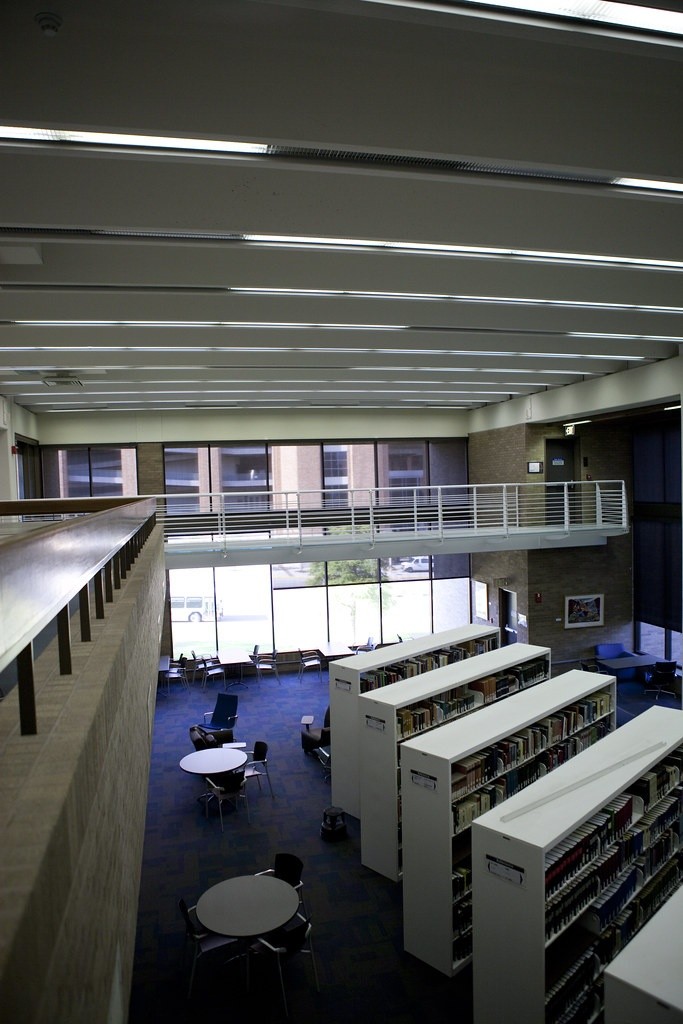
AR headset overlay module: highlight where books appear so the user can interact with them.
[545,746,683,1024]
[395,657,549,875]
[451,689,613,963]
[360,636,497,694]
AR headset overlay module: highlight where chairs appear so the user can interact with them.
[644,661,678,701]
[164,646,321,1016]
[579,661,608,675]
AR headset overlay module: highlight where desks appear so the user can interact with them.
[194,875,299,971]
[179,748,248,809]
[318,641,356,681]
[598,655,668,698]
[157,655,171,697]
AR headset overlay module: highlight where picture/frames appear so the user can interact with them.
[564,593,605,630]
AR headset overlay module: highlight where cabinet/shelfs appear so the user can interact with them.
[329,625,683,1024]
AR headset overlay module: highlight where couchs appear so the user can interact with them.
[594,642,639,682]
